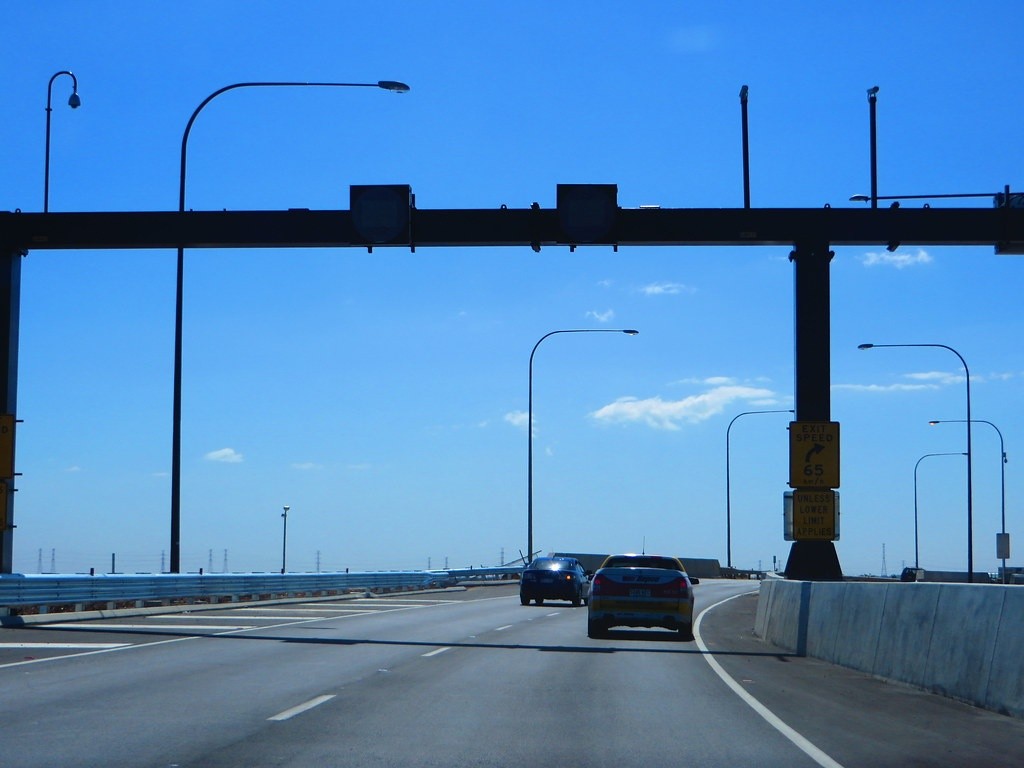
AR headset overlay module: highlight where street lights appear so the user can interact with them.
[914,452,968,568]
[528,329,639,562]
[858,344,973,584]
[169,82,411,573]
[281,506,289,575]
[929,421,1006,585]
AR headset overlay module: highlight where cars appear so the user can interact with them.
[520,557,590,607]
[587,554,699,642]
